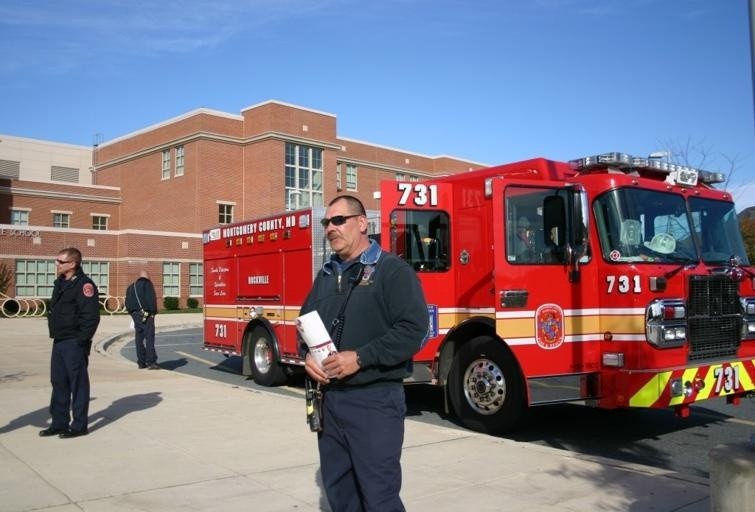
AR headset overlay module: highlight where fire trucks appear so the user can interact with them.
[202,153,755,433]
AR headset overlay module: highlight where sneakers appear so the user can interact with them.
[138,363,160,370]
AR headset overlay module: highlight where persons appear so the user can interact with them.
[296,194,431,512]
[125,269,162,369]
[39,247,101,440]
[326,344,336,357]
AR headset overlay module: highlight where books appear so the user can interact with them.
[293,310,340,380]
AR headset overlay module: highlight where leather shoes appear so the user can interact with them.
[39,426,88,439]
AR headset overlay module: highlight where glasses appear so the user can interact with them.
[57,259,72,264]
[320,215,361,228]
